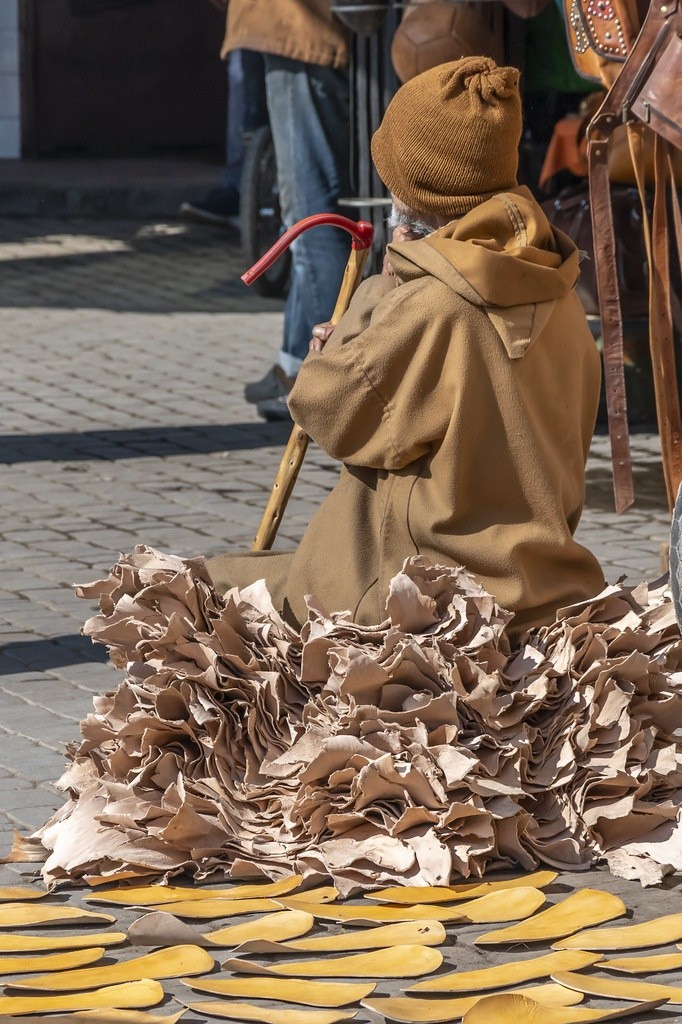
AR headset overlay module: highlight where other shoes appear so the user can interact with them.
[370,56,523,216]
[242,364,306,406]
[257,393,293,420]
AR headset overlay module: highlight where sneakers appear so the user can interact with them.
[181,186,245,225]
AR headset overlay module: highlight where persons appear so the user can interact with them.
[216,0,361,421]
[208,54,606,643]
[180,49,268,230]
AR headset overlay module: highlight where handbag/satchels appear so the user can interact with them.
[536,178,650,318]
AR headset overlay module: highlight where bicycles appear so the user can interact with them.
[239,122,293,297]
